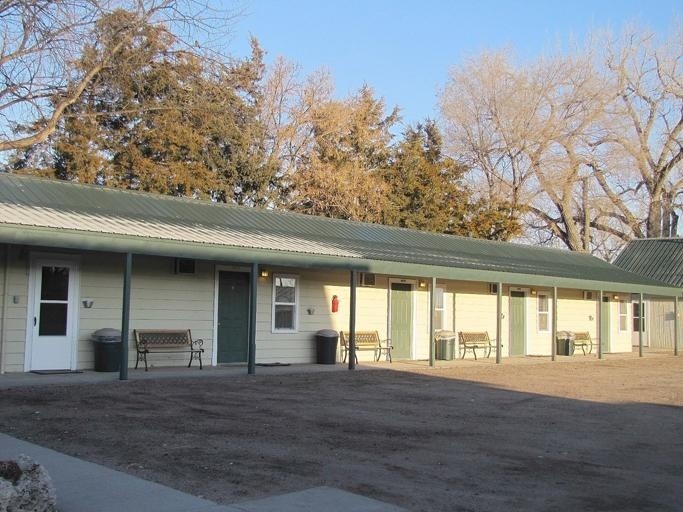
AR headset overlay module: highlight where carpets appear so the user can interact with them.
[254,361,290,367]
[28,368,84,374]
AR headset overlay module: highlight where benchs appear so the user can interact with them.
[340,330,394,363]
[459,330,504,360]
[556,331,593,355]
[133,328,206,370]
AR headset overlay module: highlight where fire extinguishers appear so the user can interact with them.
[332,295,339,312]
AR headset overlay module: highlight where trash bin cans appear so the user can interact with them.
[315,329,339,364]
[556,330,570,356]
[91,328,122,372]
[435,329,455,361]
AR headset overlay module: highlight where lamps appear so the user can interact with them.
[613,294,619,300]
[531,287,536,296]
[306,307,316,315]
[418,279,427,288]
[261,264,269,277]
[79,296,95,309]
